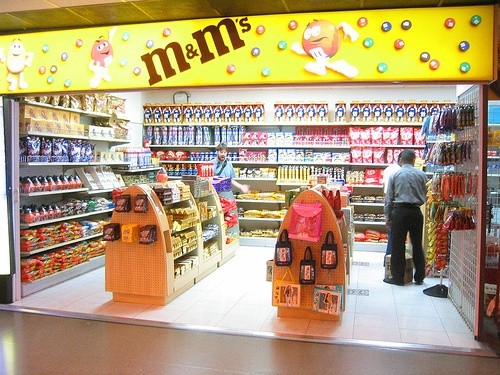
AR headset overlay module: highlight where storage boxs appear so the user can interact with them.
[75,166,125,190]
[384,252,413,285]
[95,151,125,163]
[19,104,116,137]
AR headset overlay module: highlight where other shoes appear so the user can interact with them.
[383,277,404,286]
[416,279,423,285]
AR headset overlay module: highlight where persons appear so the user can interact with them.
[383,150,428,286]
[211,143,251,233]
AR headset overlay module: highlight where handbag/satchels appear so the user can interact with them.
[299,247,315,285]
[321,231,338,268]
[276,229,292,266]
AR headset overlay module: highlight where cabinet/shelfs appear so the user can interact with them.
[103,176,242,307]
[271,181,355,322]
[140,99,455,221]
[15,100,131,299]
